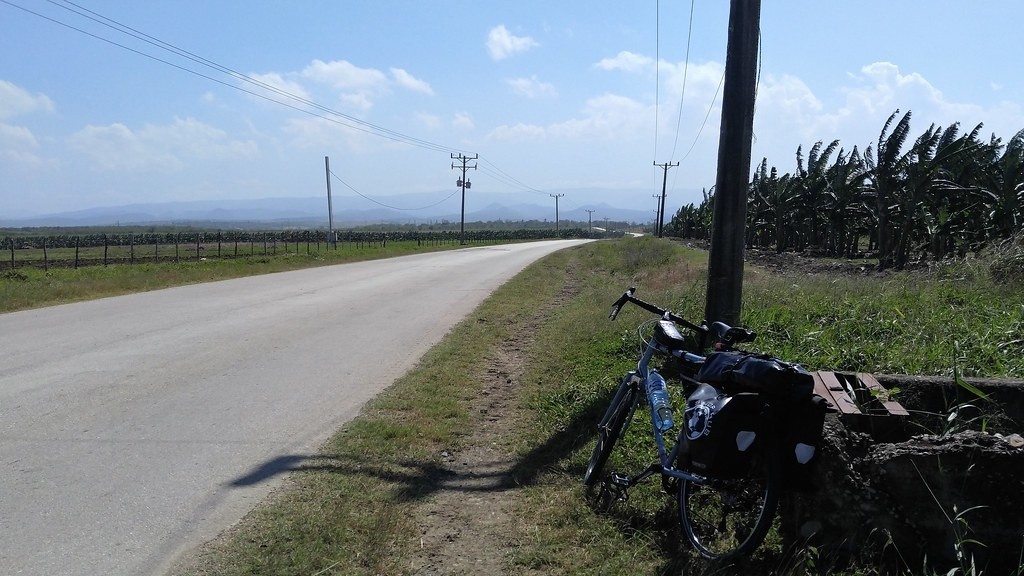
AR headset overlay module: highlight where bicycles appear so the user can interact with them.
[581,285,784,564]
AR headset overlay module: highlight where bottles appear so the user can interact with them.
[646,367,672,430]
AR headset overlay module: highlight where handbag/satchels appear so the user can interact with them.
[677,352,827,493]
